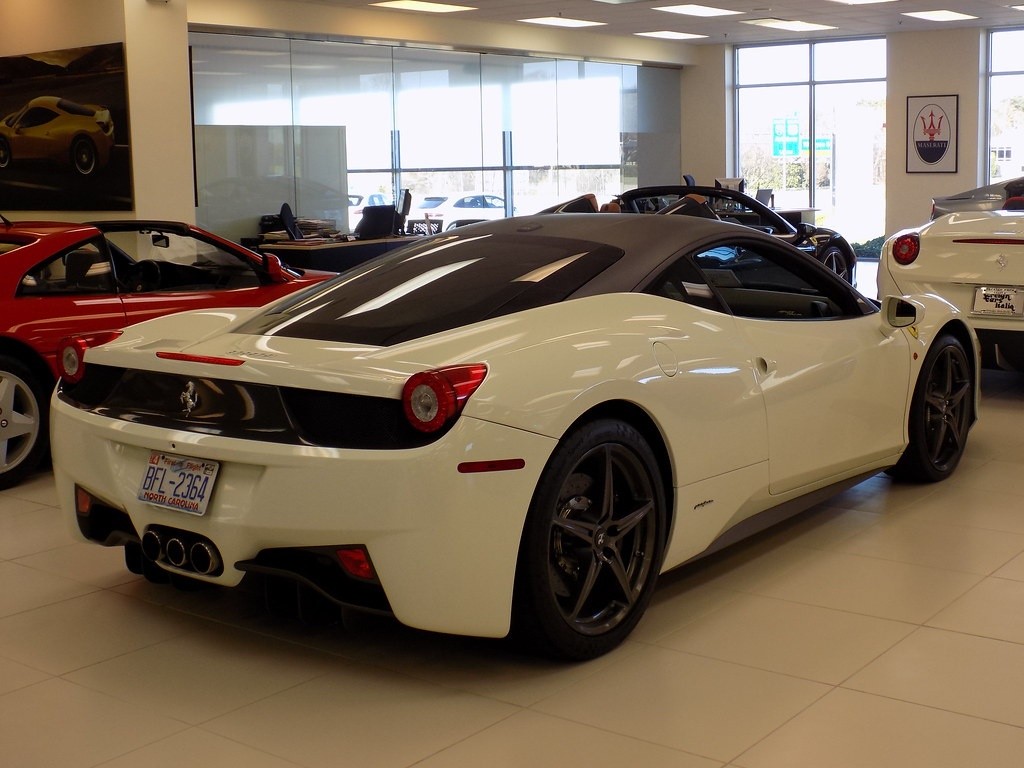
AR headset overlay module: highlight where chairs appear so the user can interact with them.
[280,202,306,240]
[354,206,403,240]
[64,249,109,296]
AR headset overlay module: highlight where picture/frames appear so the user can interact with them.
[905,94,959,174]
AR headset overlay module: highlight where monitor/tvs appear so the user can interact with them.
[397,188,415,236]
[715,178,744,212]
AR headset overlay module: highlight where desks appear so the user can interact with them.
[713,208,821,235]
[259,234,422,274]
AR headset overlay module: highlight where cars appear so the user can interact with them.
[416,193,522,232]
[337,192,393,234]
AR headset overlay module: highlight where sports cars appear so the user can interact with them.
[1,94,114,176]
[48,220,984,664]
[873,211,1024,372]
[929,175,1024,222]
[197,174,342,232]
[530,185,858,293]
[0,220,340,488]
